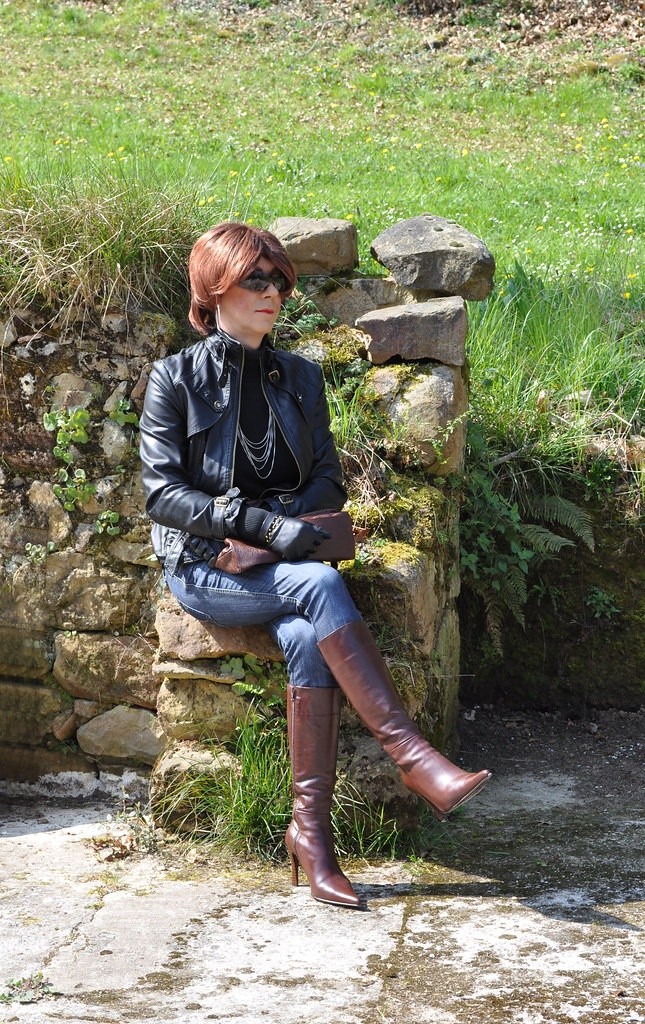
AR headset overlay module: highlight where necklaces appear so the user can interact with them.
[238,407,276,479]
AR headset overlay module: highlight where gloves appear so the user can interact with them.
[257,511,331,562]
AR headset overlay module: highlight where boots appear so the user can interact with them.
[284,682,362,907]
[318,618,492,822]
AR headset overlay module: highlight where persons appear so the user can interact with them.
[140,222,492,907]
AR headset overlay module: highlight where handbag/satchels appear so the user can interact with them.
[215,511,356,576]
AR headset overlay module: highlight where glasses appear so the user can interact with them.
[234,265,293,292]
